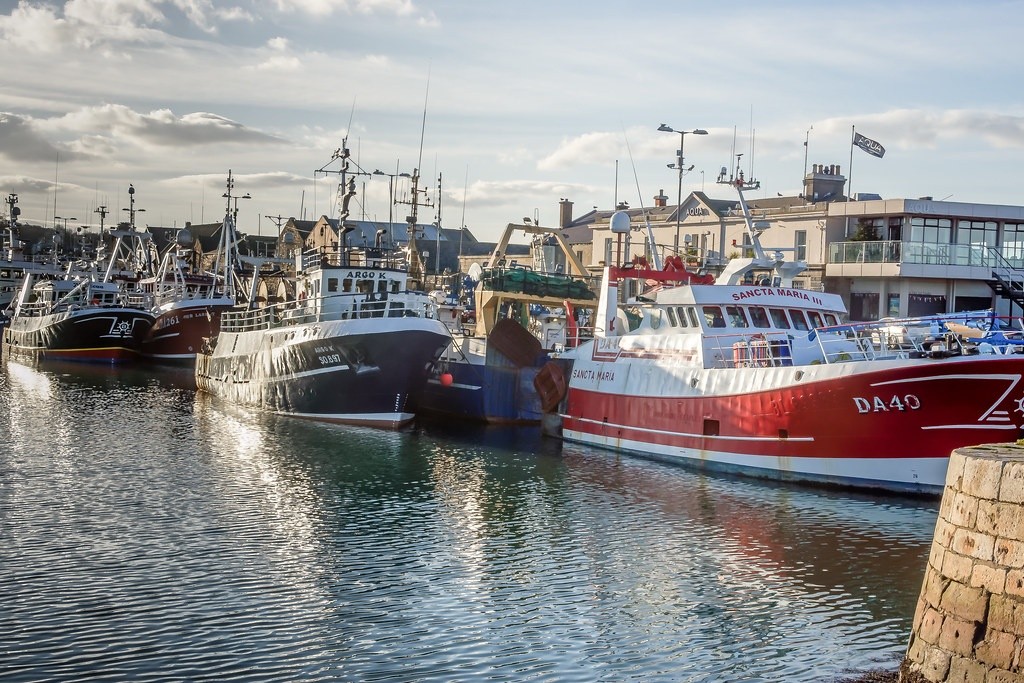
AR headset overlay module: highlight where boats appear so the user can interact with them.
[193,119,455,432]
[543,129,1024,498]
[0,152,236,367]
[413,316,570,430]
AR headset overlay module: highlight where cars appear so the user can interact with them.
[872,316,932,349]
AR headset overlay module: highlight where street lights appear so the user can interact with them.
[657,125,709,254]
[122,208,146,225]
[222,194,251,231]
[54,216,77,240]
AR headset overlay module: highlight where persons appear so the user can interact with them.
[712,308,727,328]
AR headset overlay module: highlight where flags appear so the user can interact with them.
[854,133,886,159]
